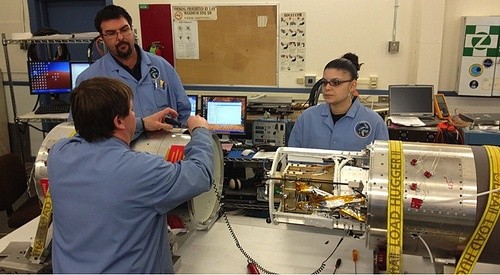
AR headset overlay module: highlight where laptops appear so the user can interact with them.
[460,113,500,126]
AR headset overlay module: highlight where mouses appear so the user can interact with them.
[241,149,255,156]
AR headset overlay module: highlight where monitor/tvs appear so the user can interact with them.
[389,84,434,118]
[201,95,247,136]
[27,60,72,94]
[187,95,198,117]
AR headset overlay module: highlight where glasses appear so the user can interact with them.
[98,24,133,37]
[320,78,357,87]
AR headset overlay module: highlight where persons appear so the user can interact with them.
[47,76,215,274]
[288,53,390,170]
[68,5,191,128]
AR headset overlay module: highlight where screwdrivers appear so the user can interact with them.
[333,259,341,275]
[469,120,475,130]
[352,249,358,274]
[164,117,188,128]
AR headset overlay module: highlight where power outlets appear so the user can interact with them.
[305,76,316,86]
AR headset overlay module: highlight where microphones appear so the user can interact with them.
[230,178,261,190]
[165,116,189,129]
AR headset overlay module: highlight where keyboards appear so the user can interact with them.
[35,104,70,114]
[423,120,438,127]
[222,143,233,151]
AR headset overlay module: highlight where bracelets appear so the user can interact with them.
[141,117,146,132]
[192,126,207,133]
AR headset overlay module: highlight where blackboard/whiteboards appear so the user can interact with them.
[173,2,280,89]
